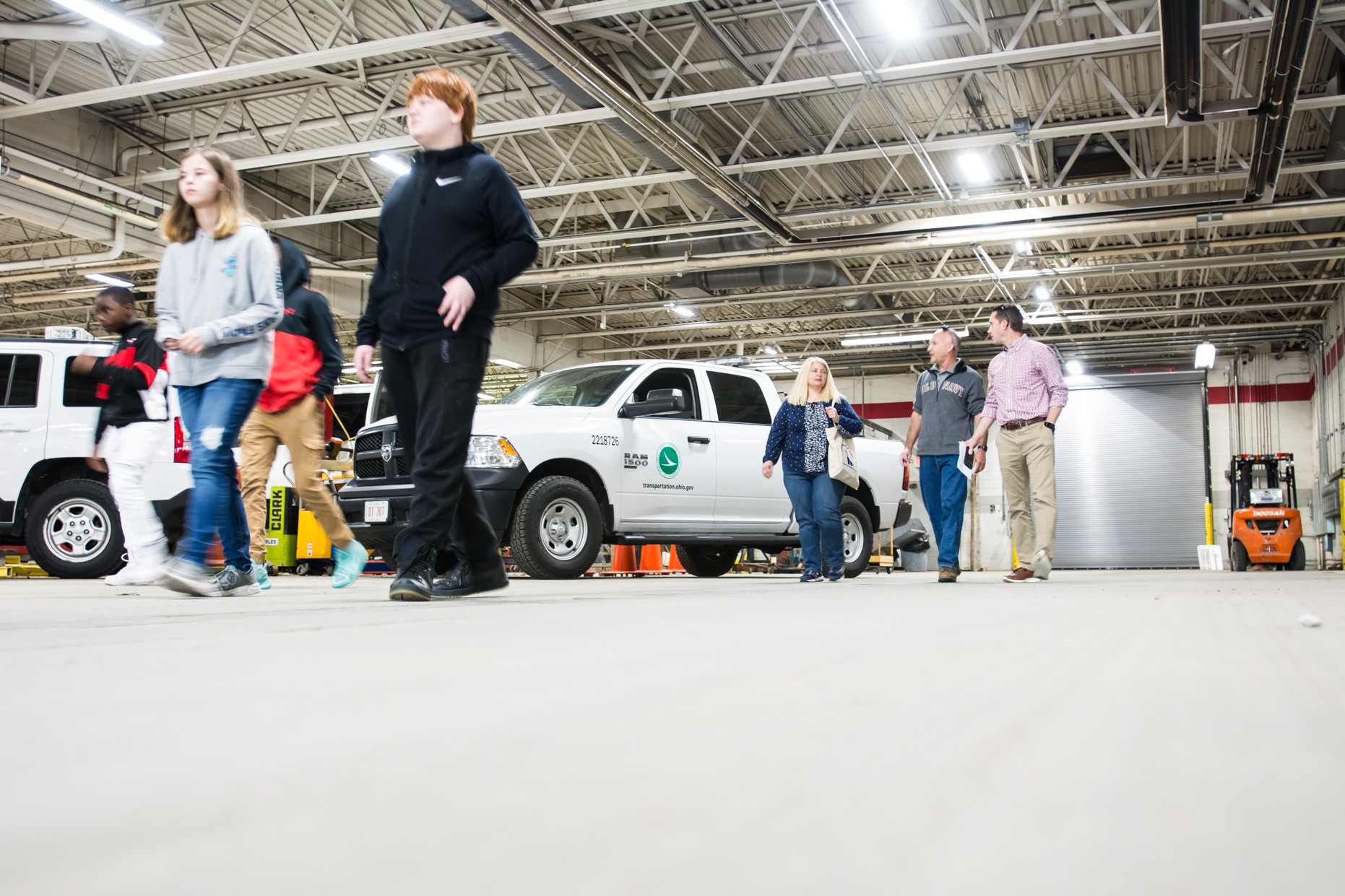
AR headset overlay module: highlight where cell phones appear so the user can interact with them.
[964,446,975,469]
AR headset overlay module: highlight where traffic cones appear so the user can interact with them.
[666,543,688,575]
[637,544,670,576]
[598,544,639,575]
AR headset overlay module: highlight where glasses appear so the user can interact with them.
[941,325,956,350]
[993,304,1010,327]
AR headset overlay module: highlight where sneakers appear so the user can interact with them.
[158,559,216,597]
[938,561,961,583]
[1032,548,1052,581]
[828,563,847,582]
[430,555,509,599]
[799,569,824,582]
[205,563,262,596]
[389,546,438,600]
[1003,566,1042,582]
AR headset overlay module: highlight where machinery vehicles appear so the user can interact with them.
[1224,452,1306,571]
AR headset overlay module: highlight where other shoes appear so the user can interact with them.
[249,559,271,590]
[332,539,369,588]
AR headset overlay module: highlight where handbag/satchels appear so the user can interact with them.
[824,425,860,491]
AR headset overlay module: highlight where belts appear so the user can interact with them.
[1000,416,1045,431]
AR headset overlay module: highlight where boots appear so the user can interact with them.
[103,536,170,585]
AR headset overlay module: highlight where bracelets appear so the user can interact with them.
[1045,422,1055,432]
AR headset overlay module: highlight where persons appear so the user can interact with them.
[238,235,369,589]
[965,305,1067,582]
[763,357,863,581]
[354,69,539,599]
[155,146,285,597]
[72,287,169,587]
[900,325,988,582]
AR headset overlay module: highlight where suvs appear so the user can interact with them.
[253,381,384,515]
[0,323,195,580]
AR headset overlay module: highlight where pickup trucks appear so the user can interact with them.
[337,359,929,581]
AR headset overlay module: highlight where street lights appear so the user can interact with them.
[1194,339,1216,545]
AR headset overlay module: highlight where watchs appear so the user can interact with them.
[978,444,988,452]
